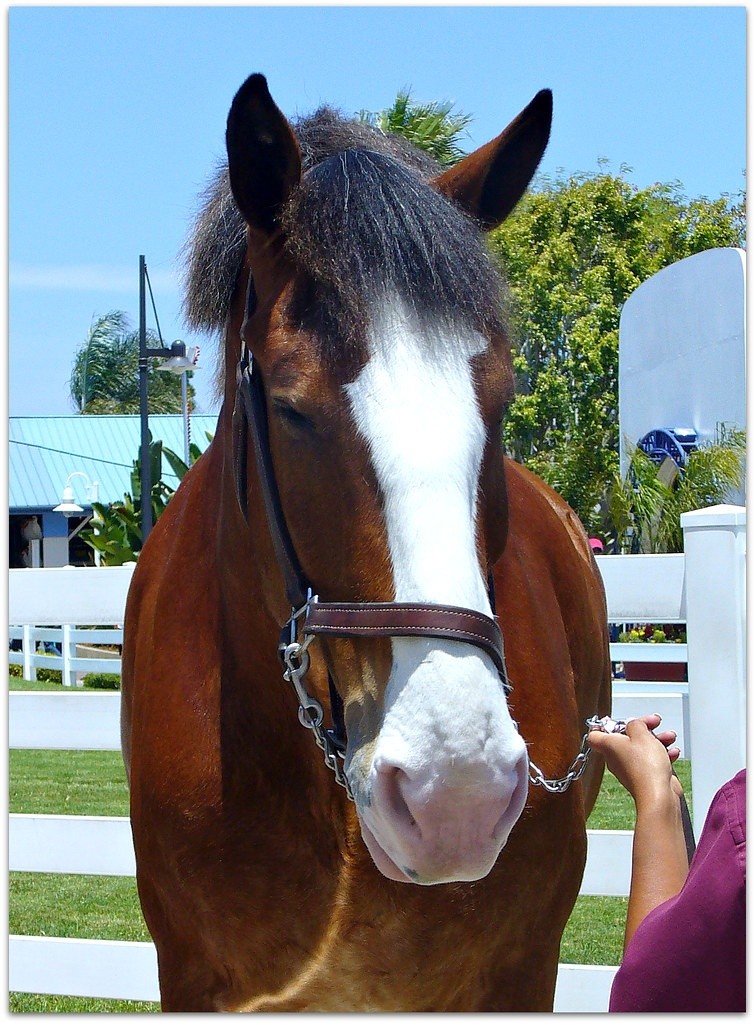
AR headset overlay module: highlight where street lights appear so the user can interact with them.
[138,254,203,548]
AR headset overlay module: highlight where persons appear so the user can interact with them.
[589,538,618,678]
[585,714,747,1017]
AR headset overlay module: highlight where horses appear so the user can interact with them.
[119,69,612,1015]
